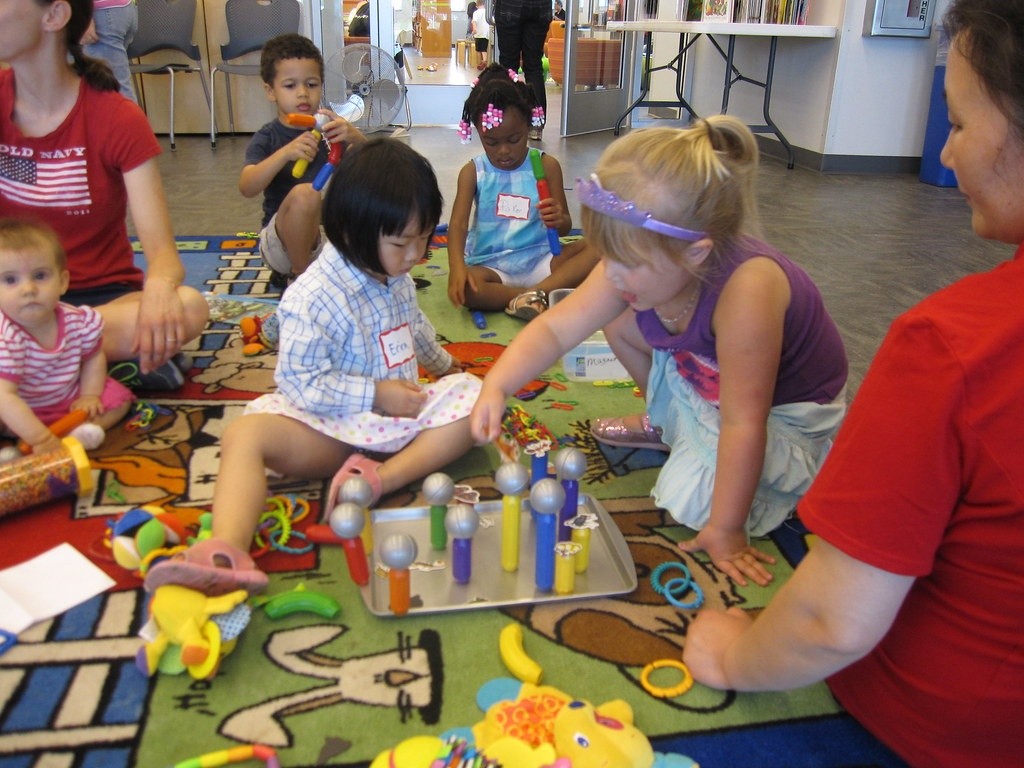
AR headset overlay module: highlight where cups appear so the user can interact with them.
[0,435,94,517]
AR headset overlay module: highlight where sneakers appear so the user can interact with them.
[108,356,187,396]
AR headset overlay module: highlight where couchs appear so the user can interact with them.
[548,20,622,91]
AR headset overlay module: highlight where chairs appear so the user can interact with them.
[395,29,416,79]
[126,0,219,152]
[210,0,300,153]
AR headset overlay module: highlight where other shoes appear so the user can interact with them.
[320,454,383,525]
[143,540,269,597]
[281,269,301,291]
[527,125,542,140]
[477,60,488,70]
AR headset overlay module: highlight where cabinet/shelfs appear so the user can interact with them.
[412,0,452,59]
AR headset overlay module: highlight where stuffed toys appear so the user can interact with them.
[368,676,655,768]
[134,544,252,680]
[518,54,550,83]
[104,503,189,579]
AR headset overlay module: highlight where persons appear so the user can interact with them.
[238,33,370,275]
[146,138,483,593]
[0,216,136,455]
[494,0,552,140]
[468,115,848,589]
[78,0,140,110]
[553,0,565,21]
[0,0,212,376]
[466,0,490,70]
[682,0,1024,768]
[447,62,605,321]
[394,39,405,84]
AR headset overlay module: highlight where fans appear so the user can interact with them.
[320,43,412,134]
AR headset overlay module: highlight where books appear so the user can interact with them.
[577,24,591,31]
[591,0,624,26]
[731,0,810,25]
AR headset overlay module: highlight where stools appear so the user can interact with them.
[456,39,481,67]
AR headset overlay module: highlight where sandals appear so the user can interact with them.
[590,411,669,451]
[503,289,548,321]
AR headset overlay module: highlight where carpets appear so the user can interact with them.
[0,228,892,768]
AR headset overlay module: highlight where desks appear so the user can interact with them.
[605,20,838,171]
